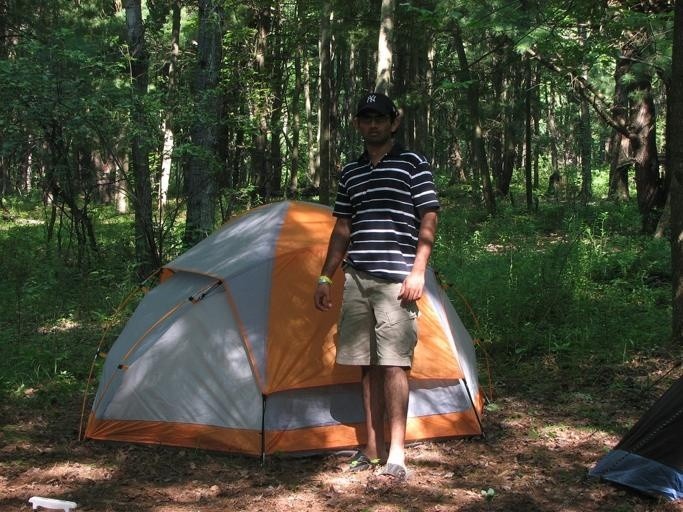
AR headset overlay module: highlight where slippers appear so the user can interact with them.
[372,463,412,485]
[334,450,385,474]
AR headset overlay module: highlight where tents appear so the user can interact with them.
[81,198,490,458]
[588,371,683,503]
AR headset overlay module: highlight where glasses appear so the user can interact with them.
[362,114,385,125]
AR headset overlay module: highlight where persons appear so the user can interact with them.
[311,91,443,495]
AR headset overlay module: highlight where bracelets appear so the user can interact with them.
[318,275,333,287]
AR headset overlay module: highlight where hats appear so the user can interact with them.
[357,93,399,120]
